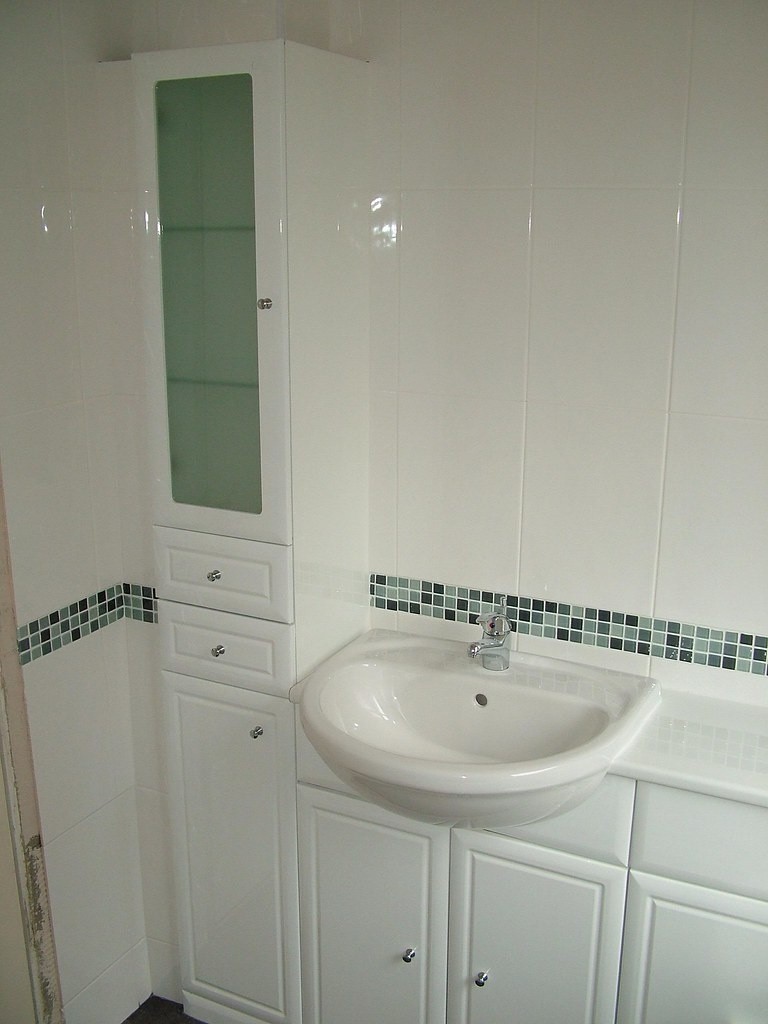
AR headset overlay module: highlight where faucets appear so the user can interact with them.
[468,611,512,671]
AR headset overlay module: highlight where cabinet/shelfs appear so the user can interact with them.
[292,703,637,1024]
[130,38,375,1024]
[615,777,767,1024]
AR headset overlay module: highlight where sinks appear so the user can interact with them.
[299,629,664,826]
